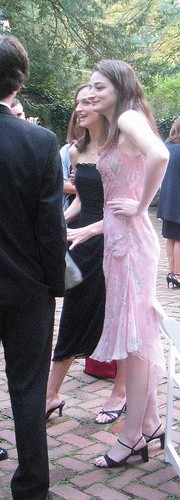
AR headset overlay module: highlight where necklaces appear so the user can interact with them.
[91,153,95,155]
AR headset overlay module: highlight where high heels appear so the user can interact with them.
[94,402,127,424]
[45,400,65,419]
[172,274,180,289]
[94,435,148,468]
[167,273,174,288]
[143,423,165,449]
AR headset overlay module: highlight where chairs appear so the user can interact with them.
[153,298,180,477]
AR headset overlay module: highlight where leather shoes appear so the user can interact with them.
[0,448,8,461]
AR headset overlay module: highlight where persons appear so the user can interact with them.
[0,35,67,500]
[59,108,84,214]
[11,98,40,123]
[157,117,180,288]
[67,61,169,466]
[45,85,126,425]
